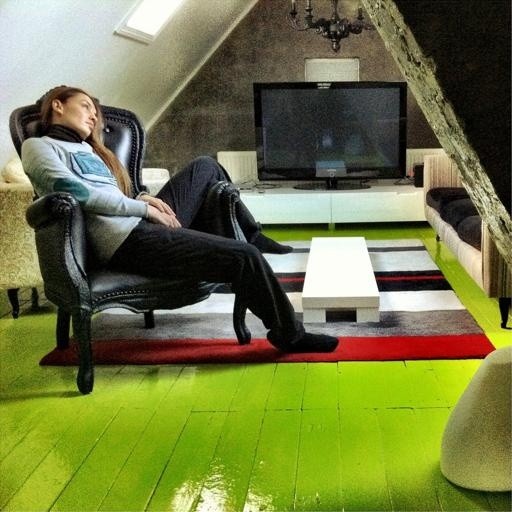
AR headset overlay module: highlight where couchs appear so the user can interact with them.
[424,149,512,328]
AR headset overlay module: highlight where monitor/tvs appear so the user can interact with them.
[253,81,408,190]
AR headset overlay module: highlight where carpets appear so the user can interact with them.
[36,236,497,365]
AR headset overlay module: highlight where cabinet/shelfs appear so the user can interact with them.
[233,178,425,231]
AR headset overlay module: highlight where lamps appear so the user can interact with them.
[285,1,375,53]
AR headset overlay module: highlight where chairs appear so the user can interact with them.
[6,105,251,395]
[2,157,50,319]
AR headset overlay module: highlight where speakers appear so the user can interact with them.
[413,162,424,187]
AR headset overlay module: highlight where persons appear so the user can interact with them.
[20,85,339,353]
